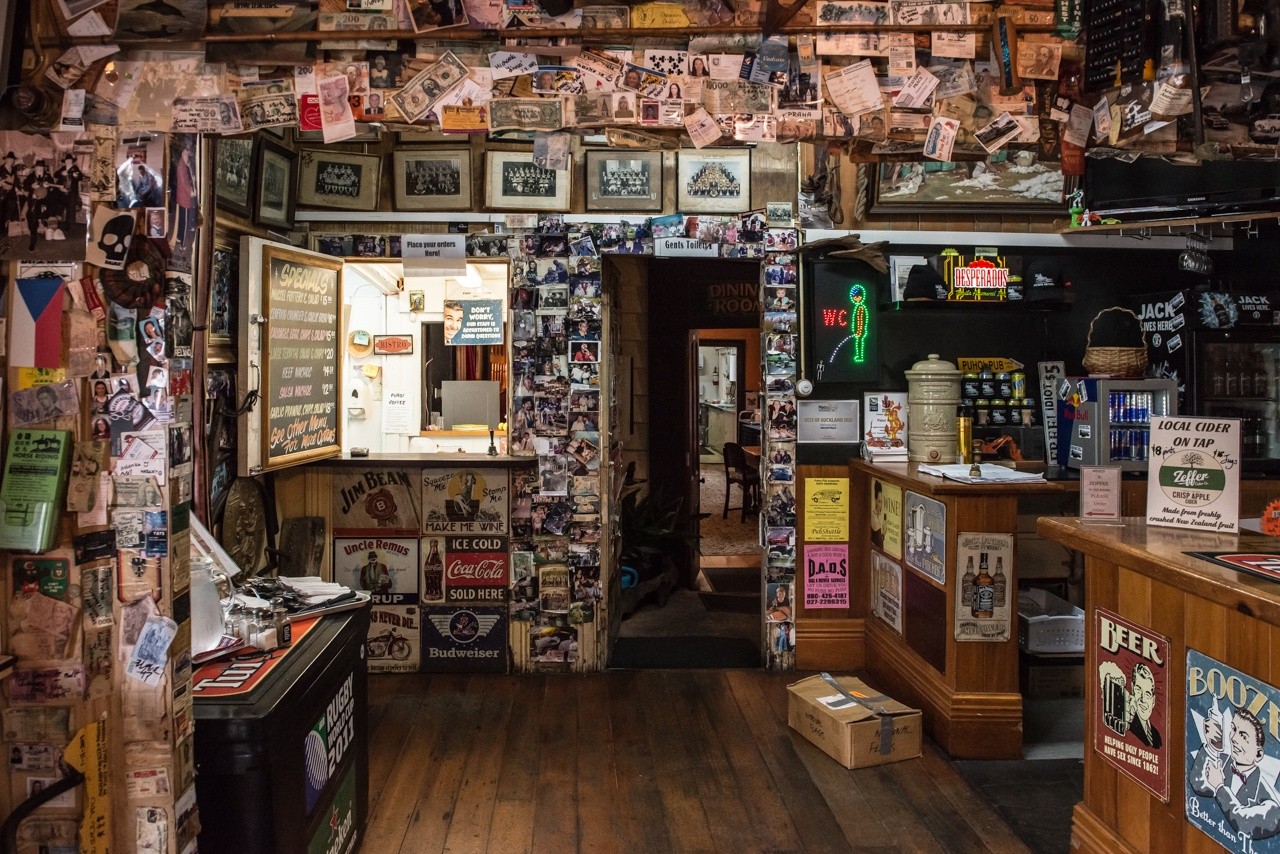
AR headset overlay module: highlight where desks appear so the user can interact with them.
[741,445,763,510]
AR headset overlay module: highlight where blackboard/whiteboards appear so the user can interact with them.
[261,244,345,469]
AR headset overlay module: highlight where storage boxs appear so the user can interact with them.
[787,672,924,772]
[929,254,1024,300]
[192,590,372,854]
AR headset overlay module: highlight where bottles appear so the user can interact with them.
[962,556,977,606]
[971,552,994,618]
[225,603,292,653]
[1209,295,1230,327]
[1205,345,1269,460]
[992,556,1006,607]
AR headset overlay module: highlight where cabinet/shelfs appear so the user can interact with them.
[707,409,736,453]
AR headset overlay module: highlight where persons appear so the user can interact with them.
[1,1,1280,854]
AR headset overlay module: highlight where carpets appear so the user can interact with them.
[952,755,1085,854]
[699,444,715,455]
[607,565,762,669]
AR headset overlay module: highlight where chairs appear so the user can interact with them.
[722,442,762,524]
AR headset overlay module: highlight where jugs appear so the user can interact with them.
[189,556,235,657]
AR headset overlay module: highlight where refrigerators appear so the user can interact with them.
[1055,376,1179,478]
[1132,284,1280,478]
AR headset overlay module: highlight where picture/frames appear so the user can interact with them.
[865,159,1080,218]
[202,229,239,364]
[480,122,753,216]
[213,126,478,231]
